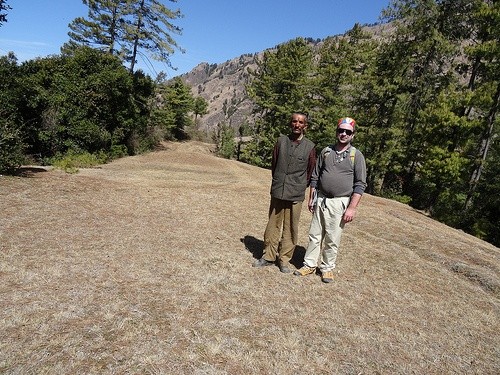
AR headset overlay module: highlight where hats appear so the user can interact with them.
[337,117,357,133]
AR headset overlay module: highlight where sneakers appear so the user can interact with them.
[292,264,317,276]
[320,270,334,283]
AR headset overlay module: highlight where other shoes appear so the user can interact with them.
[277,260,290,273]
[250,258,276,267]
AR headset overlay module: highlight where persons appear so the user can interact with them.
[252,111,316,273]
[294,117,366,283]
